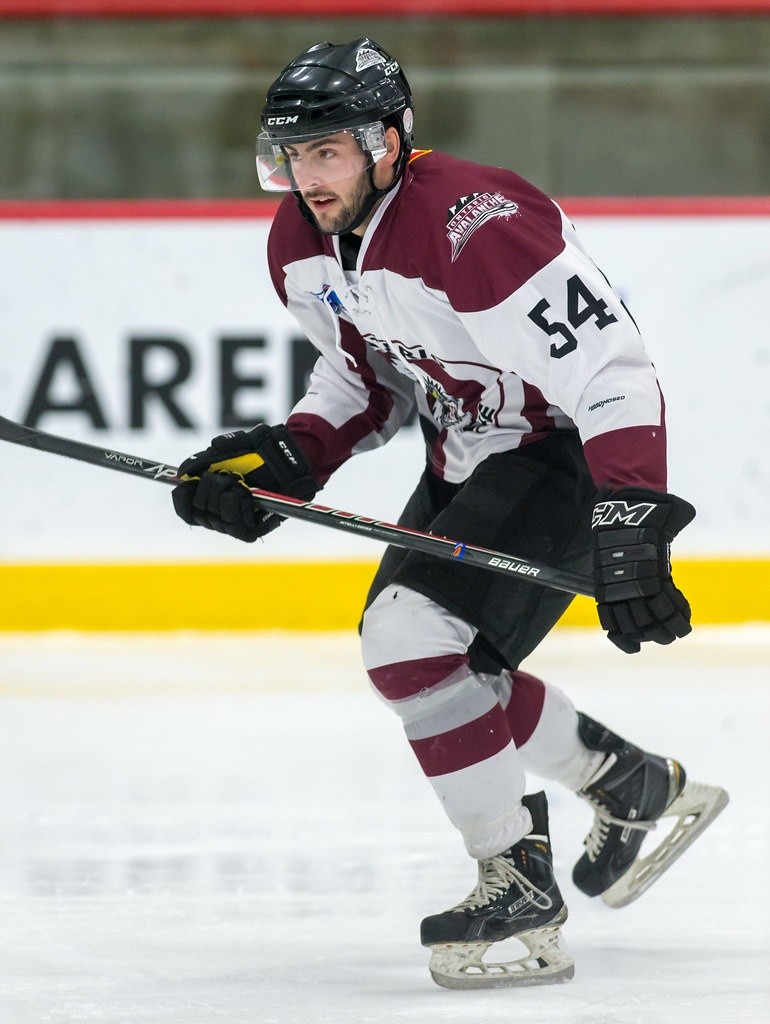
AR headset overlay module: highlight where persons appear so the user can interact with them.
[170,36,729,990]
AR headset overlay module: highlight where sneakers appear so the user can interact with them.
[568,712,729,908]
[418,793,576,985]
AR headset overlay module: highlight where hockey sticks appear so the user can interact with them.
[0,415,598,601]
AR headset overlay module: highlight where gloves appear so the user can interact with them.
[173,423,318,544]
[589,487,698,654]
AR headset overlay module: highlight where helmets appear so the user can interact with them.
[259,37,415,176]
